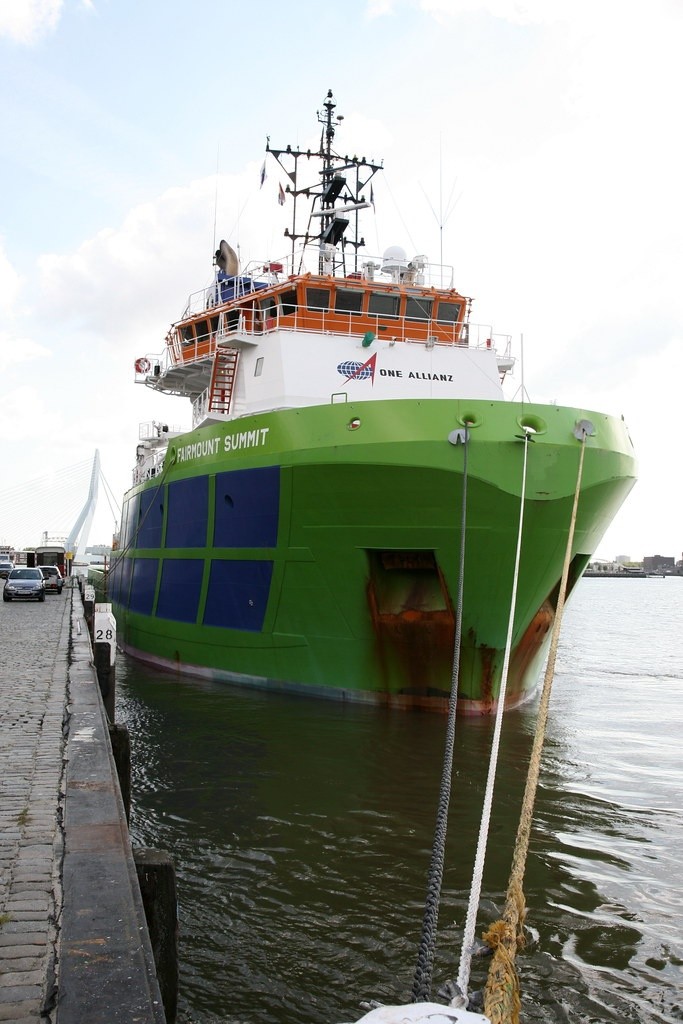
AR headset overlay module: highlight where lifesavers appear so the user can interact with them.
[134,357,151,374]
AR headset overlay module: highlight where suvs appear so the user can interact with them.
[36,565,64,593]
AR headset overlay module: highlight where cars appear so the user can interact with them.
[0,563,15,577]
[2,567,46,602]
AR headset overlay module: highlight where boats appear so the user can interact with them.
[88,82,638,719]
